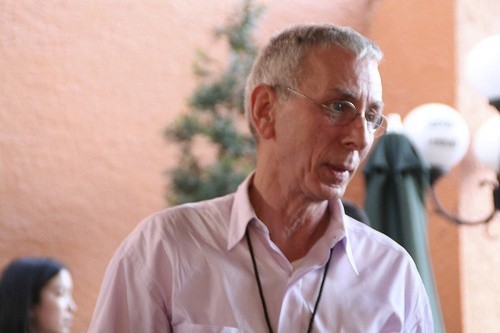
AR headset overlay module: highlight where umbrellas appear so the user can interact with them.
[362,110,446,332]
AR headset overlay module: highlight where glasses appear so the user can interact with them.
[282,87,388,137]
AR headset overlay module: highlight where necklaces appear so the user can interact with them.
[244,227,335,333]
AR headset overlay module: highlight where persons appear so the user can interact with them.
[86,20,436,332]
[0,254,76,333]
